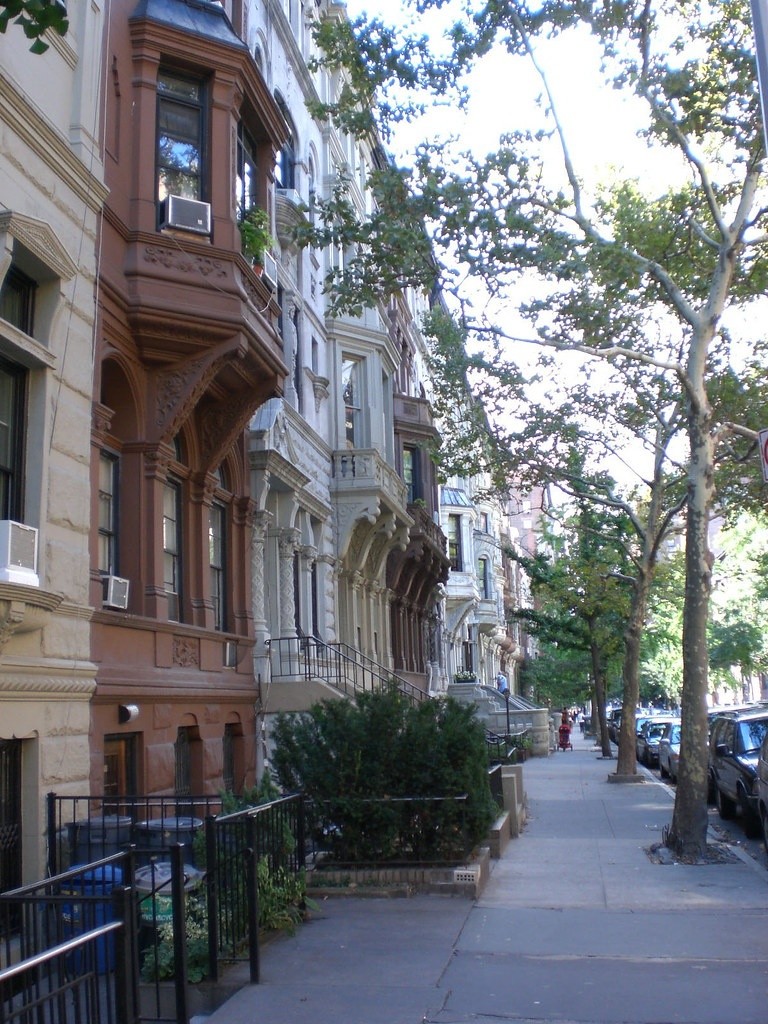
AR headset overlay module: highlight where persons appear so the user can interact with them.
[578,711,585,732]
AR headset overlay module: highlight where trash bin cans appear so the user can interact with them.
[131,863,208,981]
[52,865,122,976]
[131,817,207,868]
[67,816,131,864]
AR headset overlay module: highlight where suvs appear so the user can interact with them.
[757,731,768,853]
[709,708,768,823]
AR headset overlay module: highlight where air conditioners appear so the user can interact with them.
[277,189,301,206]
[264,250,277,288]
[98,575,129,610]
[159,194,212,236]
[0,520,38,575]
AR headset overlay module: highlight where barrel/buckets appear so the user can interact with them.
[136,817,204,868]
[65,815,132,882]
[59,864,122,978]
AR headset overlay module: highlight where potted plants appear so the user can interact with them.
[509,734,532,763]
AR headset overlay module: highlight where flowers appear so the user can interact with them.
[456,671,477,679]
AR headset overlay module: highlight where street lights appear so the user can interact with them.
[503,688,511,734]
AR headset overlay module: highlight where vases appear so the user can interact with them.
[457,679,475,683]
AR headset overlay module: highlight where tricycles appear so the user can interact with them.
[556,725,573,751]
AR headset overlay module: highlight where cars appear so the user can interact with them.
[658,721,710,784]
[636,719,672,765]
[608,708,672,753]
